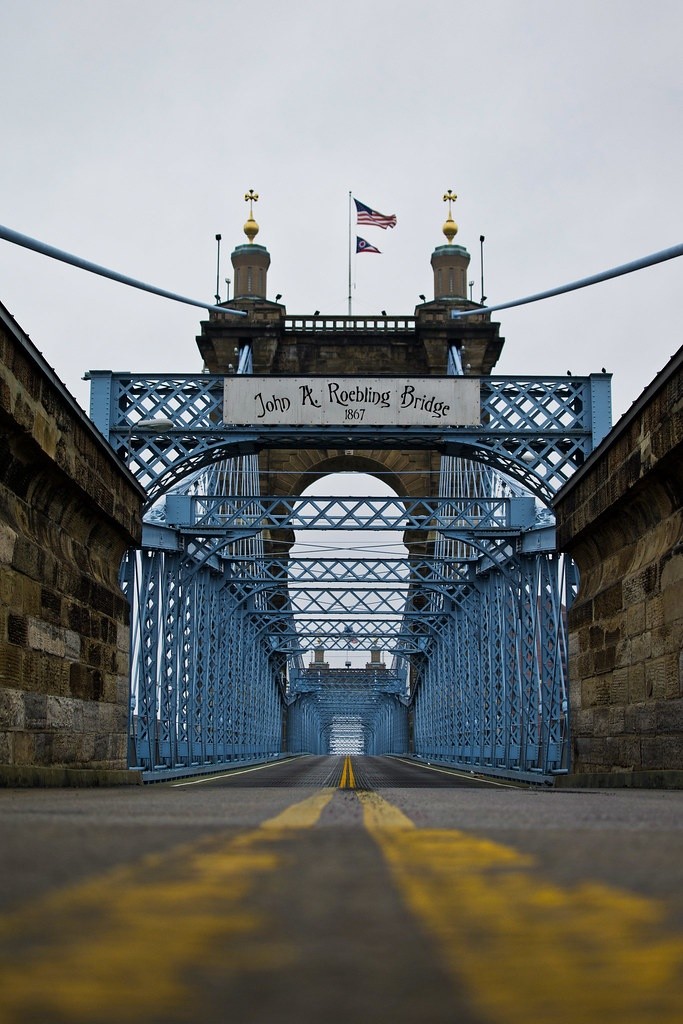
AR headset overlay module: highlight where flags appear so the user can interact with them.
[356,236,381,254]
[353,198,397,229]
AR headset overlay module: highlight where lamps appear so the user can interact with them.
[314,310,320,316]
[275,294,282,303]
[382,310,386,315]
[225,278,231,301]
[215,294,222,303]
[419,295,426,303]
[480,296,487,305]
